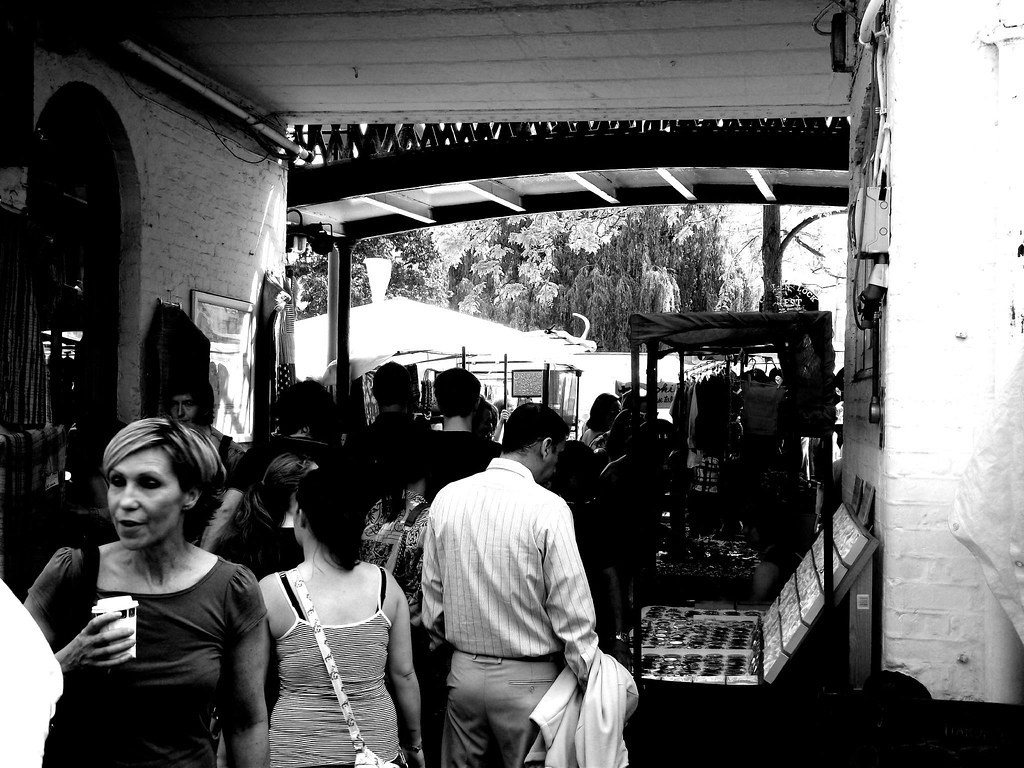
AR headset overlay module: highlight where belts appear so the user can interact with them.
[457,649,557,663]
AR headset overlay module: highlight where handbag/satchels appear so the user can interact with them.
[43,545,112,768]
[355,747,398,768]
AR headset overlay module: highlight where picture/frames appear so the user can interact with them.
[189,287,260,445]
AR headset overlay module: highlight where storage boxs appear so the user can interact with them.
[626,504,880,686]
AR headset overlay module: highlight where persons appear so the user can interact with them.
[216,466,427,768]
[420,402,639,768]
[732,516,786,607]
[22,417,269,768]
[199,361,677,721]
[156,376,245,561]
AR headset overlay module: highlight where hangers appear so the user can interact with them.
[678,360,726,383]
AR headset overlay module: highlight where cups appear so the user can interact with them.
[91,595,139,660]
